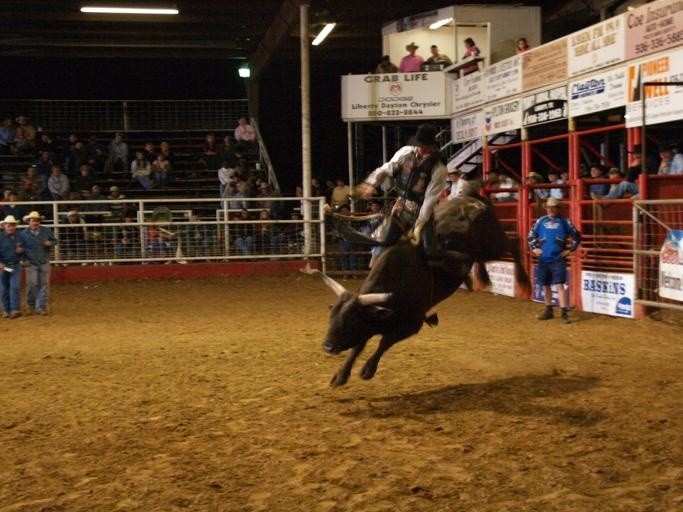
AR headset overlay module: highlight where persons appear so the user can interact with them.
[0,214,32,318]
[525,195,580,325]
[445,142,682,205]
[356,122,448,265]
[14,211,56,317]
[374,55,400,74]
[514,37,533,53]
[461,37,482,76]
[400,41,425,72]
[422,45,453,71]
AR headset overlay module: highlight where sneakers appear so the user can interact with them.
[1,307,49,320]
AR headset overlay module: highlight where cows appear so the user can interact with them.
[298,173,532,391]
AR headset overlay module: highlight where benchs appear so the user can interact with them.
[1,129,265,223]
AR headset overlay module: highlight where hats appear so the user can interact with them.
[0,214,21,229]
[524,172,544,180]
[227,169,273,200]
[627,143,680,156]
[22,210,46,224]
[589,161,623,175]
[543,197,563,210]
[407,123,440,147]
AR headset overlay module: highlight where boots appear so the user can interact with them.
[560,307,572,324]
[537,304,554,320]
